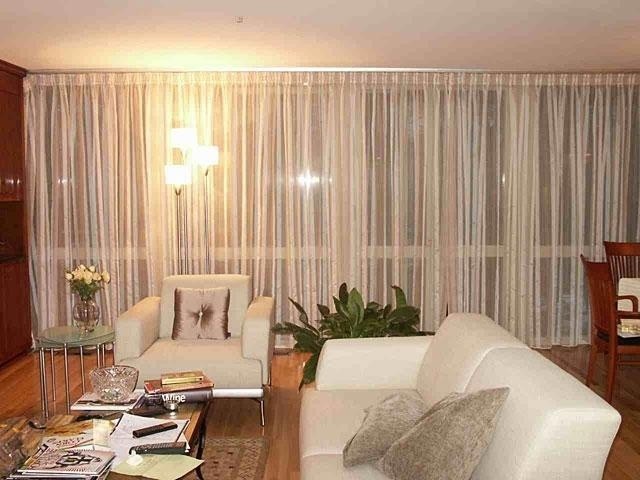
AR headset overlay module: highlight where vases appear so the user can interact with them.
[72,301,100,332]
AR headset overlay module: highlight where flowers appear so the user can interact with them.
[64,264,111,301]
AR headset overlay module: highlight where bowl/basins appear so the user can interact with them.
[88,365,139,403]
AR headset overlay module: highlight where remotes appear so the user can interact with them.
[129,441,186,455]
[132,421,178,438]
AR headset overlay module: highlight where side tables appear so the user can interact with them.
[37,325,115,418]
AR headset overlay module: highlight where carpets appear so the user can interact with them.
[182,437,269,480]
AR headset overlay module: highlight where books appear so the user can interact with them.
[8,371,214,480]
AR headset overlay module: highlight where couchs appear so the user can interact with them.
[114,274,276,427]
[297,311,622,480]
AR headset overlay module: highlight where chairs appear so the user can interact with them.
[580,241,639,402]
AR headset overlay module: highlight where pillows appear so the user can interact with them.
[171,287,230,340]
[372,386,510,480]
[342,389,430,469]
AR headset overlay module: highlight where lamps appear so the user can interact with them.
[164,127,220,196]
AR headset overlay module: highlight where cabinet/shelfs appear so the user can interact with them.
[0,59,34,367]
